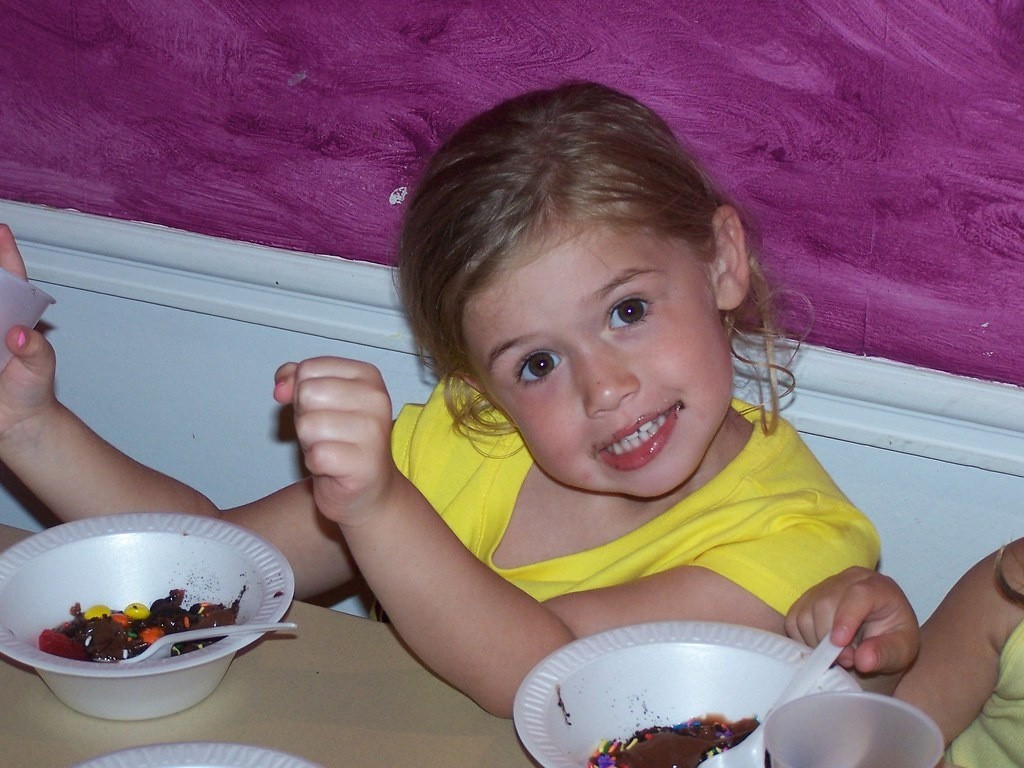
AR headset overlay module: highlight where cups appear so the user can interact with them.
[764,690,944,768]
[0,268,57,375]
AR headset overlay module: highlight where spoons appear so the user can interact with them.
[93,622,297,665]
[699,630,845,768]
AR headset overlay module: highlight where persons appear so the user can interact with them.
[784,536,1024,767]
[0,77,882,719]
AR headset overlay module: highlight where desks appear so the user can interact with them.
[0,526,948,768]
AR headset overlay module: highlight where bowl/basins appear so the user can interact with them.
[512,622,864,768]
[0,511,295,722]
[75,741,318,768]
